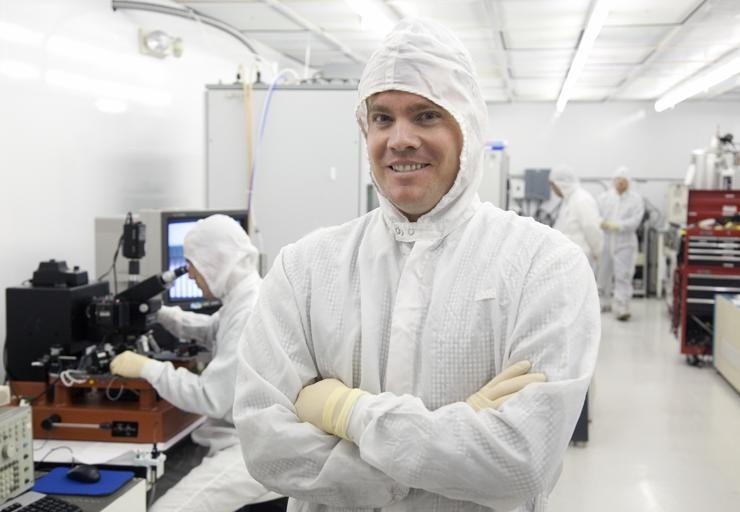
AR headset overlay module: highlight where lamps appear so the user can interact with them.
[135,28,186,58]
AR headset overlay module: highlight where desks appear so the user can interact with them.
[1,407,207,512]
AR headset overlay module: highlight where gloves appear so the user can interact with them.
[110,350,151,380]
[464,359,548,412]
[294,378,366,441]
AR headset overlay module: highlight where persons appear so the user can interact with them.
[596,167,645,320]
[110,214,288,512]
[549,167,604,267]
[233,17,601,512]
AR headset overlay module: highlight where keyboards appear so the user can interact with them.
[0,490,84,512]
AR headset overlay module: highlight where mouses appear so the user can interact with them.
[66,466,98,483]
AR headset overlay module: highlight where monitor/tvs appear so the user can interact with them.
[93,211,249,312]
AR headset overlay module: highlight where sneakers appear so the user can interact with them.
[617,309,630,321]
